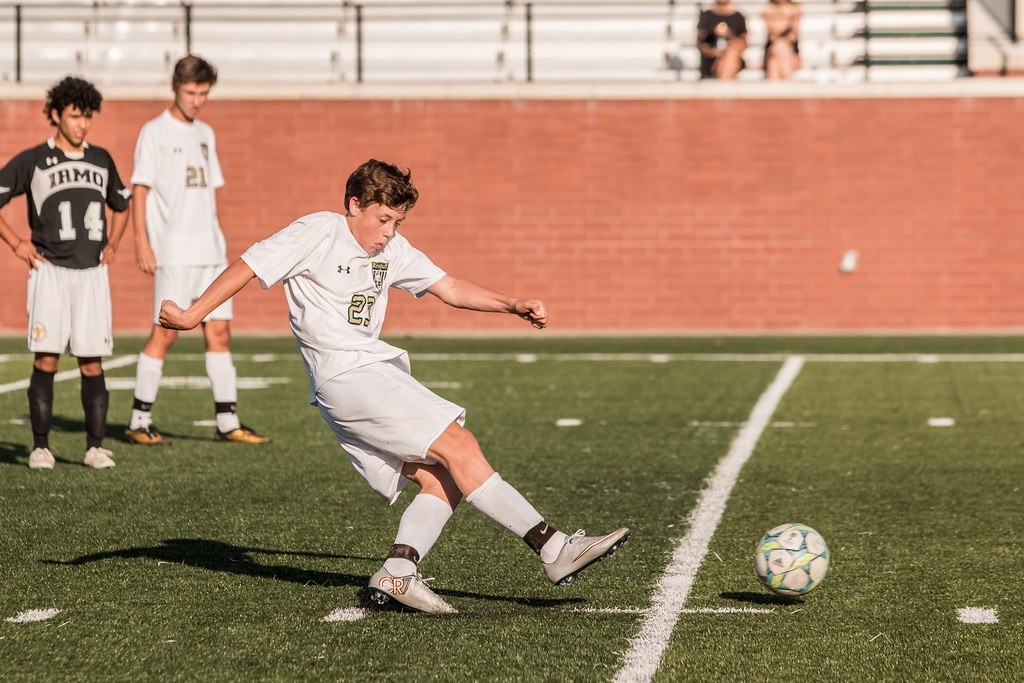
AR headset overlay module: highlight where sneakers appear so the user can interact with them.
[30,448,55,469]
[84,446,115,469]
[368,567,459,614]
[124,424,173,446]
[213,424,272,444]
[541,527,631,586]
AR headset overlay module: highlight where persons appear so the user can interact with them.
[0,75,130,470]
[697,1,747,80]
[159,158,631,614]
[760,1,802,80]
[125,54,275,446]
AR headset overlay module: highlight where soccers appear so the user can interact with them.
[751,521,833,599]
